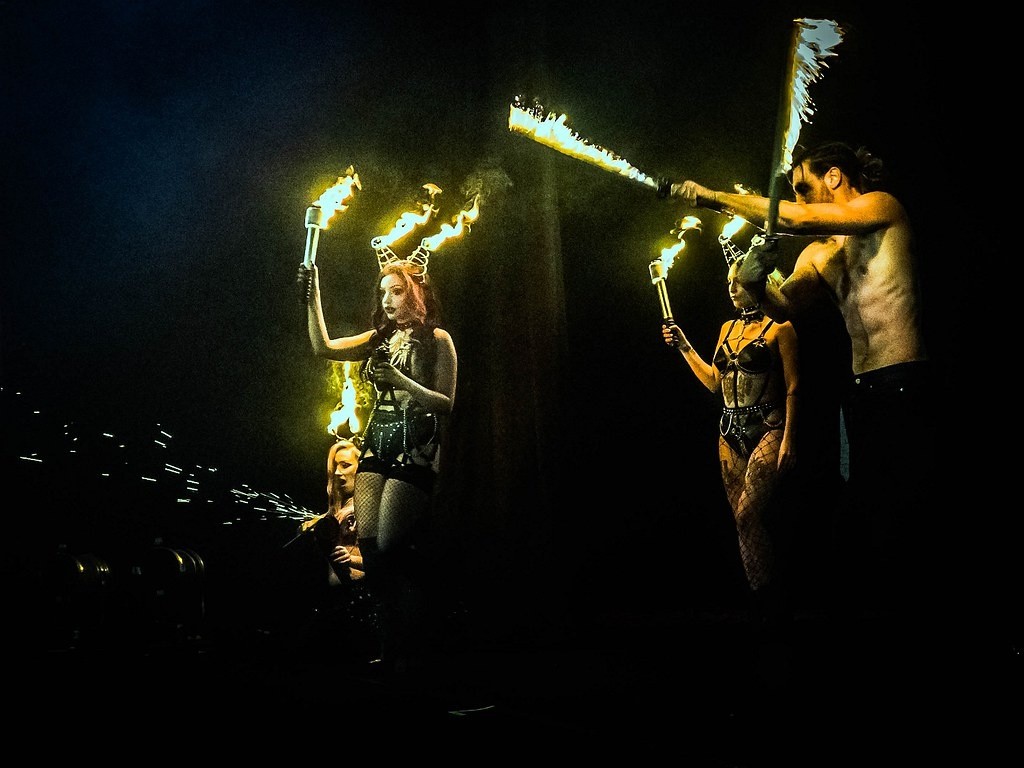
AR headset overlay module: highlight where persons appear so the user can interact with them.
[676,142,941,618]
[297,260,458,668]
[296,440,381,633]
[662,259,804,628]
